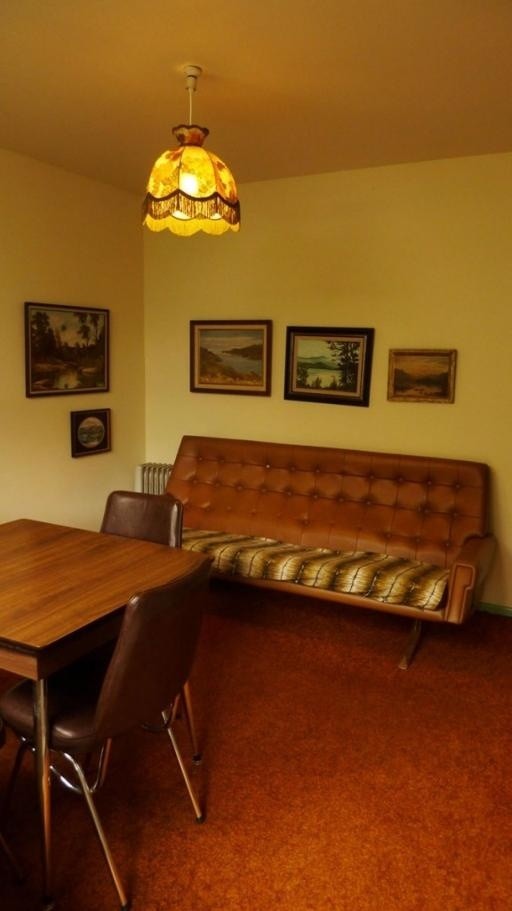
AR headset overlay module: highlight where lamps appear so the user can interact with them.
[132,64,246,240]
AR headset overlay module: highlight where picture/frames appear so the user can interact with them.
[69,408,111,458]
[22,302,111,399]
[187,320,459,406]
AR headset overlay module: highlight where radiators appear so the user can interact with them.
[137,460,175,495]
